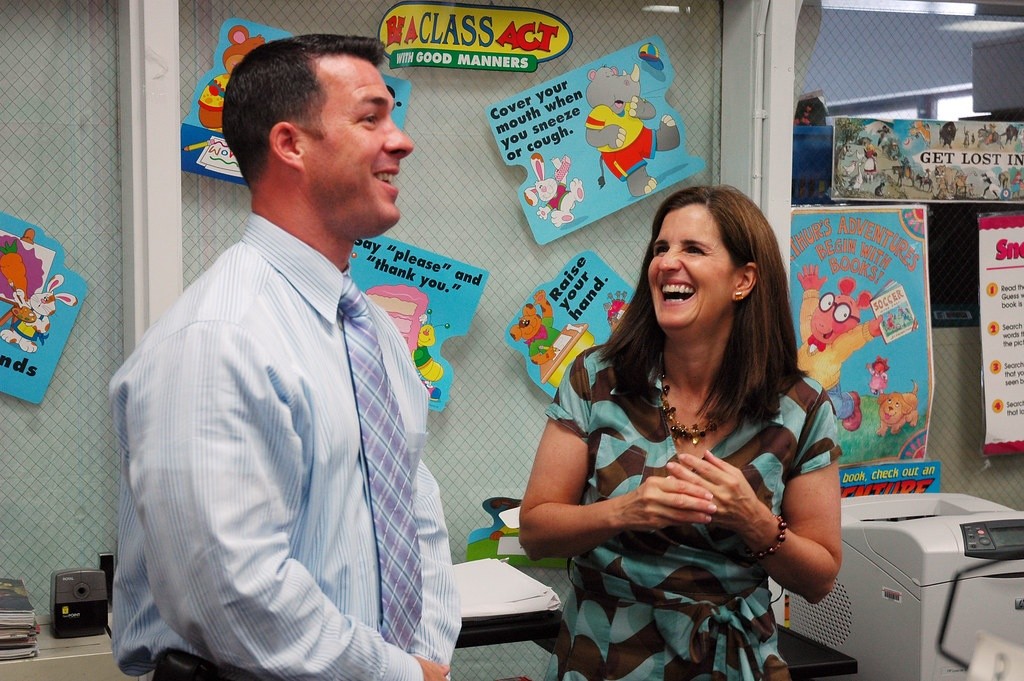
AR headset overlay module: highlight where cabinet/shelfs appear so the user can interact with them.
[1,611,146,680]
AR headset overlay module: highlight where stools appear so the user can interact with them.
[454,558,573,681]
[777,624,860,681]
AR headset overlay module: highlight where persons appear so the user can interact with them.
[111,35,472,680]
[518,186,843,681]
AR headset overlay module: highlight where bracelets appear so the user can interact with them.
[746,514,789,560]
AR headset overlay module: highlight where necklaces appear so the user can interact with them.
[656,370,737,447]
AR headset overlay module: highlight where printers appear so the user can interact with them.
[769,492,1024,680]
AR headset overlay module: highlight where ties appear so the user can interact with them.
[337,285,421,650]
